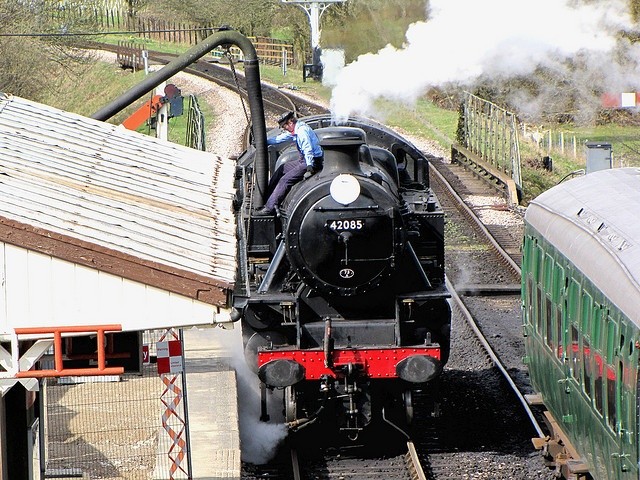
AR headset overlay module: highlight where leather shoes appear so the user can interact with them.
[252,207,271,215]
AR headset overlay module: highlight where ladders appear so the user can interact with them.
[0,324,124,379]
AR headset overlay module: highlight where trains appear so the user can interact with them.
[228,113,452,440]
[519,165,640,480]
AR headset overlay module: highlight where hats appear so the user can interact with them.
[277,111,294,128]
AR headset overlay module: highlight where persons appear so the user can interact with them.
[252,110,324,215]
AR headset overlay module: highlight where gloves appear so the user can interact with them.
[303,165,314,179]
